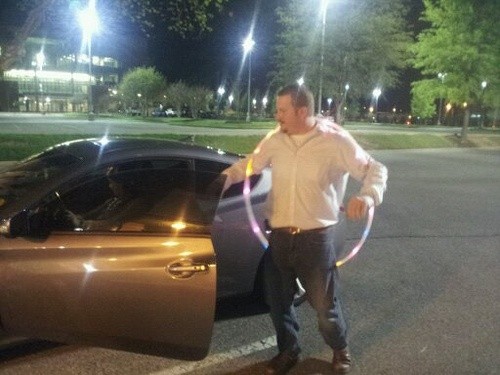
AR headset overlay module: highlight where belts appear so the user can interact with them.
[276,226,325,235]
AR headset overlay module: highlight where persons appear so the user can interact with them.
[205,83,388,375]
[64,159,170,231]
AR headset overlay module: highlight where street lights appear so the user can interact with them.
[437,72,447,126]
[246,38,255,122]
[36,54,46,115]
[316,0,329,117]
[83,12,98,121]
[373,88,382,119]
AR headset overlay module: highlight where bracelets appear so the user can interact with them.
[80,219,83,225]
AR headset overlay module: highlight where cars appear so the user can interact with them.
[1,136,350,363]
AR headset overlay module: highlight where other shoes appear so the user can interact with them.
[263,352,299,375]
[331,345,351,373]
